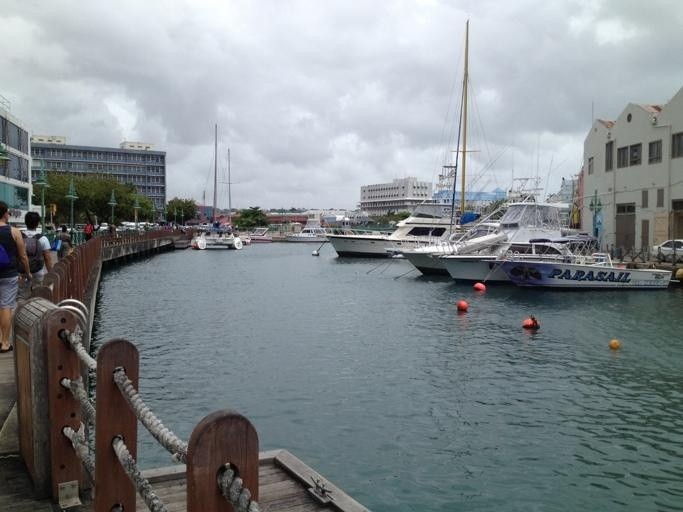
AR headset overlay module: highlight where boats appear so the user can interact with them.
[271,19,611,285]
[485,240,673,289]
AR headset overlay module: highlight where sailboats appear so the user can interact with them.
[189,123,277,249]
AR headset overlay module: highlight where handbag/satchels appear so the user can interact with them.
[49,240,62,251]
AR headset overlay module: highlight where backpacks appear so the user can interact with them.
[17,231,43,274]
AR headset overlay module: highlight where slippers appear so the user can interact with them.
[0,343,13,353]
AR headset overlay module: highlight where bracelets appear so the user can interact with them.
[26,272,30,275]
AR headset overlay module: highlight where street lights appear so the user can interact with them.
[150,201,186,230]
[64,177,79,247]
[33,155,50,236]
[131,195,141,232]
[106,188,120,238]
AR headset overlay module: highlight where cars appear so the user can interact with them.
[649,238,683,263]
[15,219,189,254]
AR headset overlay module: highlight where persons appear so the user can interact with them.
[16,211,52,307]
[83,220,94,242]
[0,201,33,353]
[56,225,73,261]
